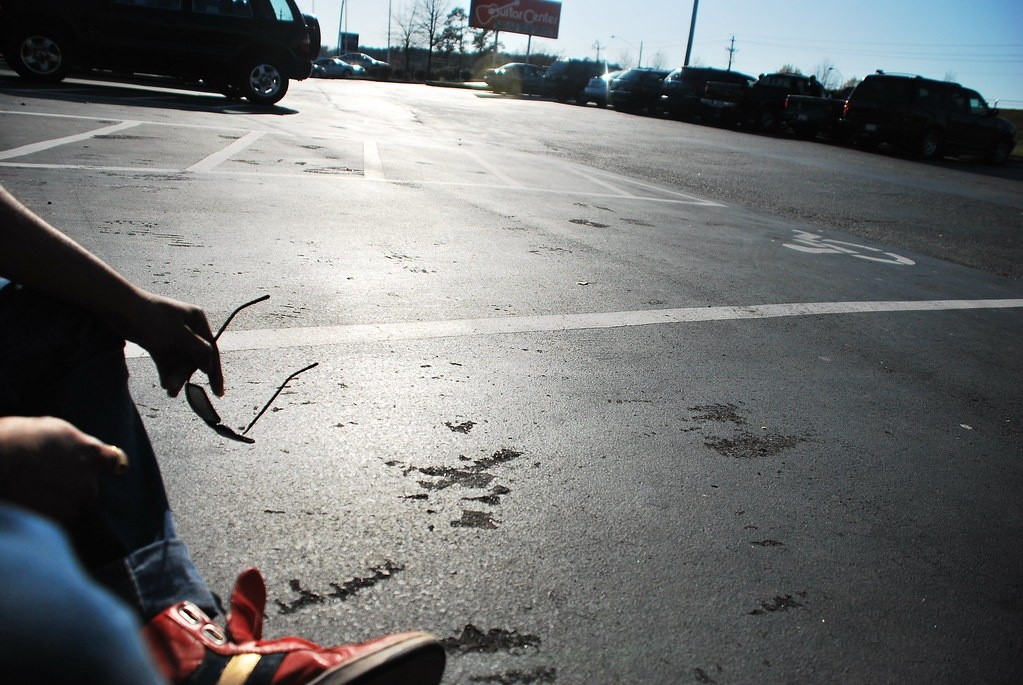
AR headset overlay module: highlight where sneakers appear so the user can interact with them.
[143,569,447,685]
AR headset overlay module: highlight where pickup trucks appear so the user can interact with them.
[699,73,829,138]
[780,86,856,143]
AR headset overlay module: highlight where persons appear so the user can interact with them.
[0,186,447,685]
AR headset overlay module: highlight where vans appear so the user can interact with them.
[611,66,671,115]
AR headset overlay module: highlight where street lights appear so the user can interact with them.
[610,34,643,68]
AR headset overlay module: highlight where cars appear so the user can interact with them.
[311,52,390,81]
[584,69,628,112]
[484,62,538,95]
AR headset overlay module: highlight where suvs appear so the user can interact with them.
[837,70,1016,166]
[657,66,757,128]
[538,58,608,108]
[0,0,321,105]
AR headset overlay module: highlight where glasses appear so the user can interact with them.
[185,295,319,444]
